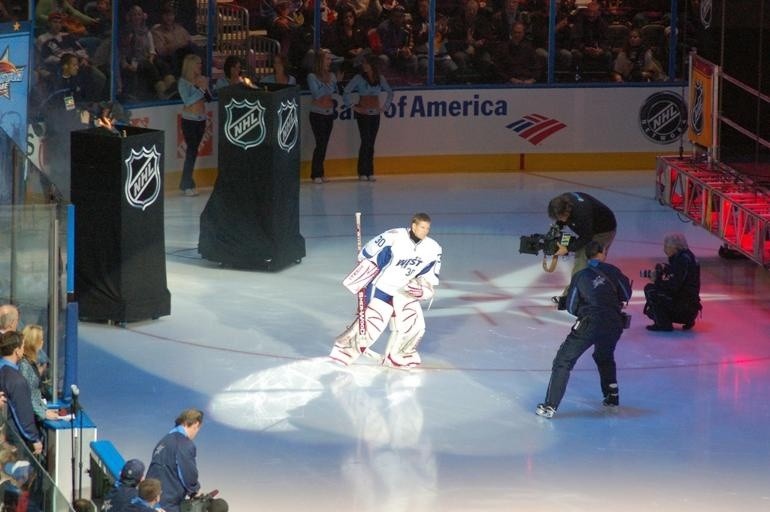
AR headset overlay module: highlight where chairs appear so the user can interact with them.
[32,0,691,103]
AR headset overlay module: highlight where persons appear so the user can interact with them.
[335,214,442,357]
[644,233,703,331]
[332,357,438,512]
[343,55,393,181]
[178,55,213,196]
[307,48,338,184]
[537,242,631,412]
[548,192,616,302]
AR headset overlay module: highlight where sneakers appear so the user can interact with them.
[602,383,619,406]
[646,323,673,331]
[537,403,558,414]
[683,311,699,328]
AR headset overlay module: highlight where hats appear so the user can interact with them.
[5,459,34,477]
[122,459,144,482]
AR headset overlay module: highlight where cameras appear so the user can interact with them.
[640,264,662,281]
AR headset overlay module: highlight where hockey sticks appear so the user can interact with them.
[356,212,384,361]
